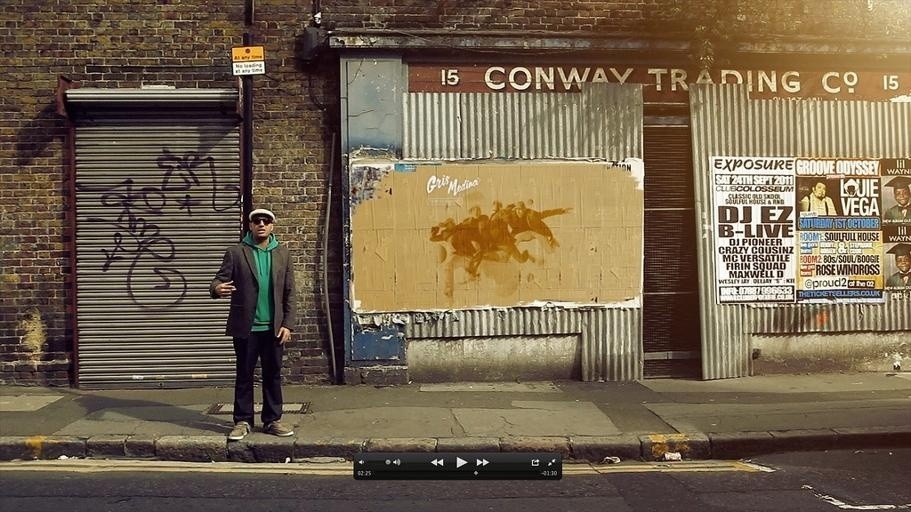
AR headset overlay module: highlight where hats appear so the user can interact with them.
[249,209,275,222]
[515,202,525,209]
[887,244,911,256]
[884,177,911,188]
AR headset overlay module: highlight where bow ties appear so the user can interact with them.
[898,205,911,212]
[900,272,911,279]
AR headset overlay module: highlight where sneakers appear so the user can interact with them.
[228,421,251,440]
[263,421,294,437]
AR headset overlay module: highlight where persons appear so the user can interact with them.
[430,200,574,295]
[884,186,911,220]
[801,182,837,216]
[209,209,297,440]
[886,254,911,287]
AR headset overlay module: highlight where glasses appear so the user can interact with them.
[250,216,273,225]
